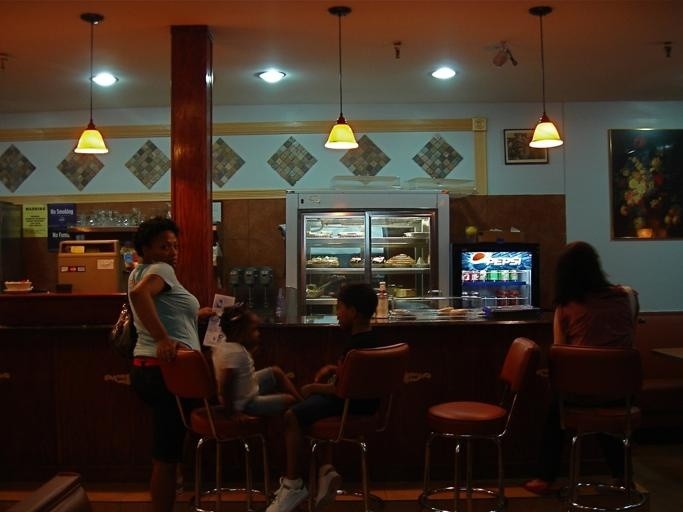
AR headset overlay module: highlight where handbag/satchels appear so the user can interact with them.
[104,305,136,356]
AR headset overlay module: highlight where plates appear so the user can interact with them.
[3,288,34,294]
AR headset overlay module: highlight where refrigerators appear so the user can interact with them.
[446,243,542,311]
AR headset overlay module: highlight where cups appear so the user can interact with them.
[81,207,166,225]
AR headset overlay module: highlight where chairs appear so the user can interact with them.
[551,338,648,512]
[407,335,542,512]
[282,342,406,511]
[154,346,271,509]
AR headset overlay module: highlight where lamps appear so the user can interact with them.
[526,4,564,150]
[322,6,359,150]
[72,12,108,156]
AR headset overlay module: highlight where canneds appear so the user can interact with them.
[462,269,519,282]
[498,288,520,306]
[461,290,480,309]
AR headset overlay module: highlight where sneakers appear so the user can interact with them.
[315,468,341,510]
[265,477,309,511]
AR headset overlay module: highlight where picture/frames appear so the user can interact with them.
[502,128,549,165]
[606,126,682,241]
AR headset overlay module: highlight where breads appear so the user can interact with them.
[435,306,453,316]
[449,307,469,320]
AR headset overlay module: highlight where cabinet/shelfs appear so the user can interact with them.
[287,193,450,313]
[458,245,538,311]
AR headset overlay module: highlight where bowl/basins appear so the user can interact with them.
[4,282,31,290]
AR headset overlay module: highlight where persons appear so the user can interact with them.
[265,279,386,512]
[126,213,219,511]
[211,303,304,417]
[524,239,642,495]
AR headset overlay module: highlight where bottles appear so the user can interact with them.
[375,281,388,318]
